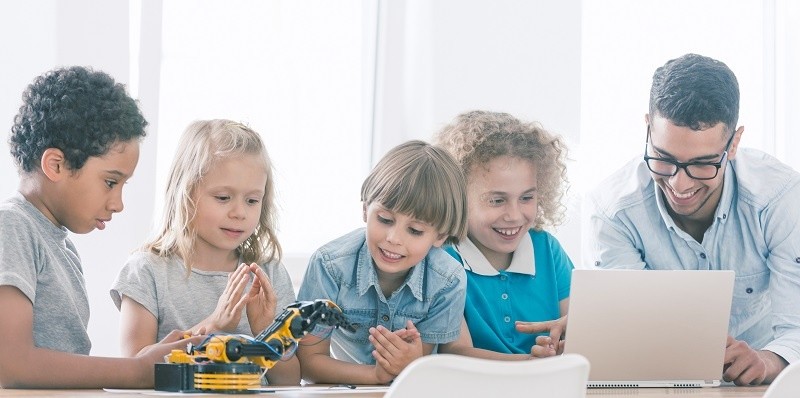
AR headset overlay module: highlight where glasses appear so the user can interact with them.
[643,123,734,180]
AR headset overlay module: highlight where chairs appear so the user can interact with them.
[382,353,589,398]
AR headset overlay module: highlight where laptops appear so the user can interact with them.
[565,268,735,388]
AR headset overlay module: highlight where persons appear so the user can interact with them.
[515,52,800,383]
[111,118,302,387]
[296,140,468,384]
[0,65,209,389]
[438,108,577,362]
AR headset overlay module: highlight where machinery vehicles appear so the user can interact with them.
[154,300,356,394]
